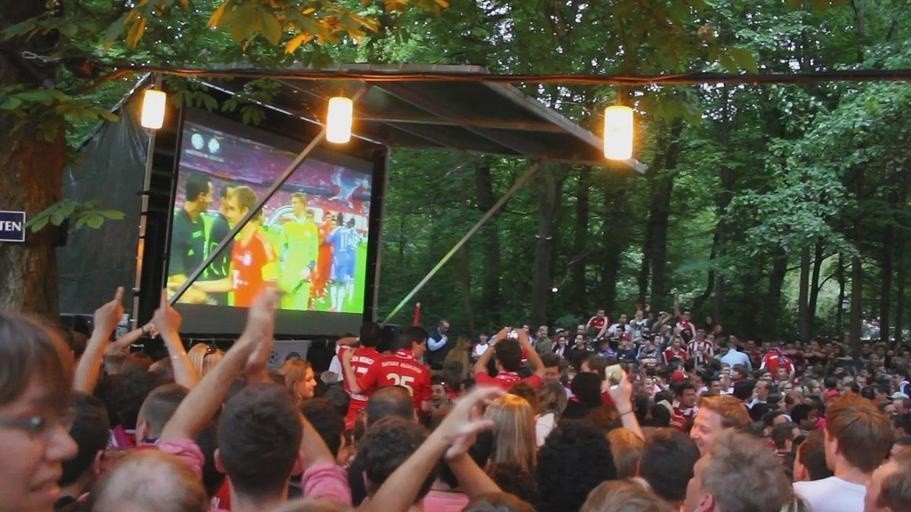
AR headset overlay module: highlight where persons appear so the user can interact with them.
[168,178,360,311]
[0,284,911,511]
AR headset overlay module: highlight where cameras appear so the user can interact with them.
[506,331,519,340]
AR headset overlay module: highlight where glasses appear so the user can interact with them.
[0,406,78,436]
